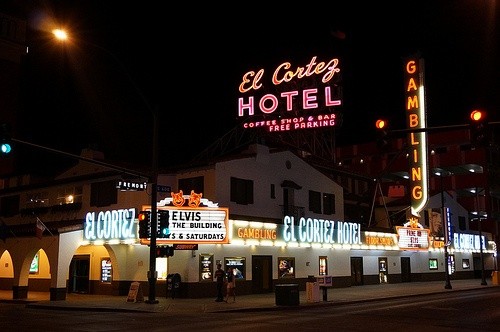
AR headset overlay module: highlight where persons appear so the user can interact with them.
[214,264,225,302]
[224,267,236,303]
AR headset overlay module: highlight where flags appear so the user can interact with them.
[35,217,45,240]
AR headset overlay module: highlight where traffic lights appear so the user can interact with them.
[137,210,152,238]
[1,125,13,156]
[375,119,388,146]
[158,210,172,238]
[470,109,486,145]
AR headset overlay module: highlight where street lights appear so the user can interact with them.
[51,27,160,304]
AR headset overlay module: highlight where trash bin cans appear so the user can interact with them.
[166,273,182,299]
